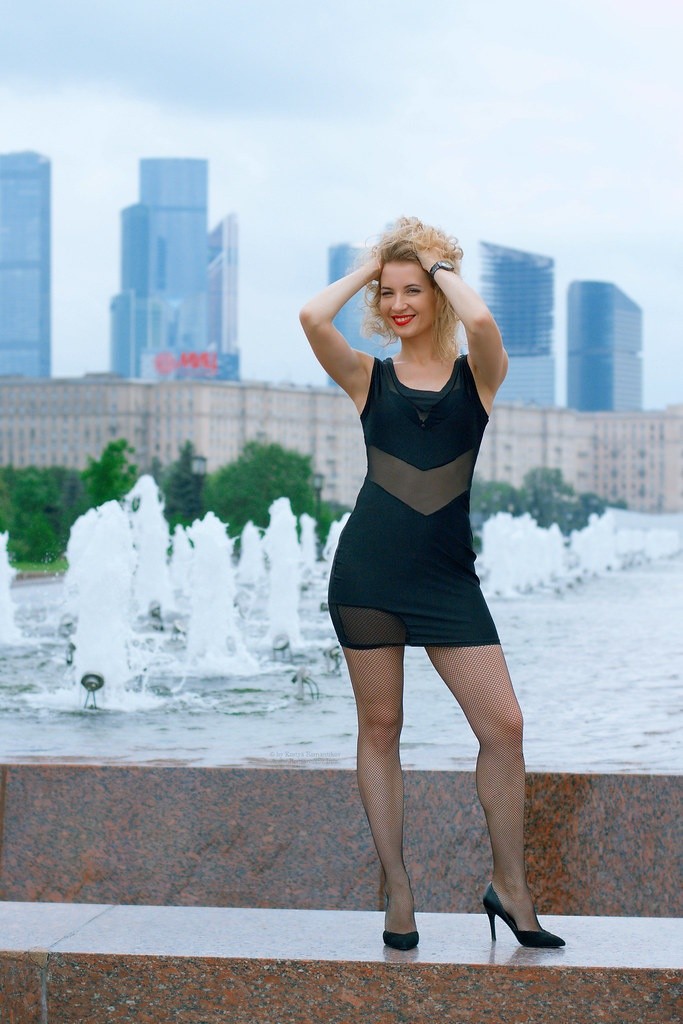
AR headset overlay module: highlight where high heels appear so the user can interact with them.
[483,880,566,948]
[383,878,419,950]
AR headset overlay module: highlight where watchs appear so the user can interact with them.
[429,261,454,278]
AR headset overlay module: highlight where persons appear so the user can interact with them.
[299,216,566,951]
